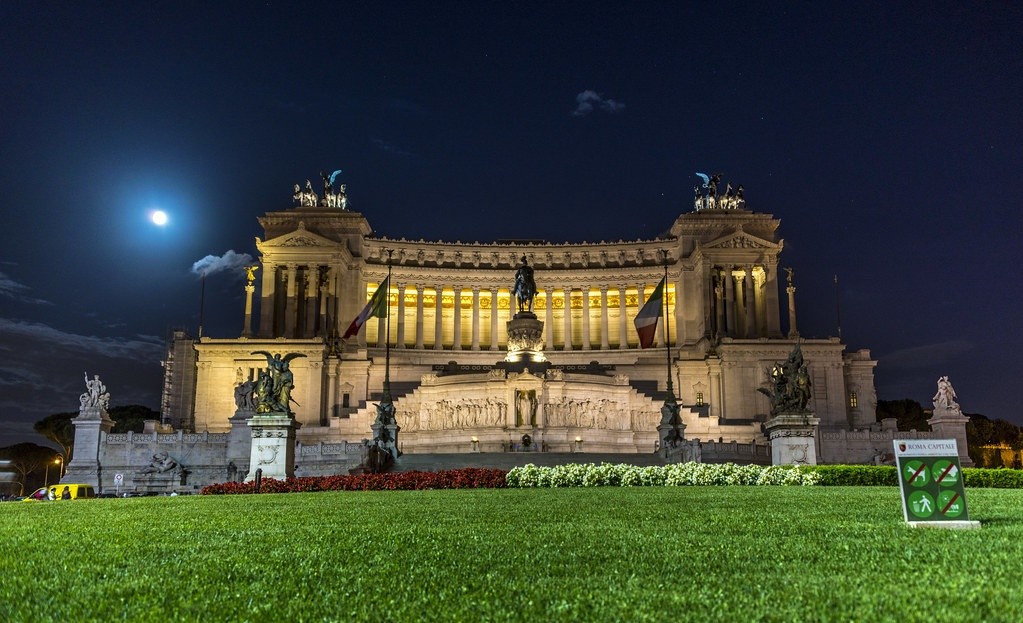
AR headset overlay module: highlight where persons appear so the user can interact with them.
[510,255,539,295]
[932,376,960,414]
[761,341,814,414]
[233,352,294,413]
[77,375,109,410]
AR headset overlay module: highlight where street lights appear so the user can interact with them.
[148,209,208,339]
[54,453,63,484]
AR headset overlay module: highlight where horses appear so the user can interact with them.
[516,268,538,313]
[292,175,353,211]
[692,182,746,210]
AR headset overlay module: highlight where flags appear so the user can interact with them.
[633,275,665,349]
[339,273,386,339]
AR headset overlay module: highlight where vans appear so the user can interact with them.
[20,484,95,504]
